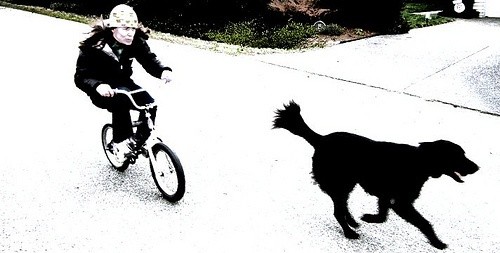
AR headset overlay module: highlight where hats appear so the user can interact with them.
[109,4,138,28]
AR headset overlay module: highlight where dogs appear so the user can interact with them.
[270,99,480,249]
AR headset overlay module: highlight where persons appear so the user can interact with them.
[74,3,174,163]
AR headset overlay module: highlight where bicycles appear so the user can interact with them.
[101,78,185,201]
